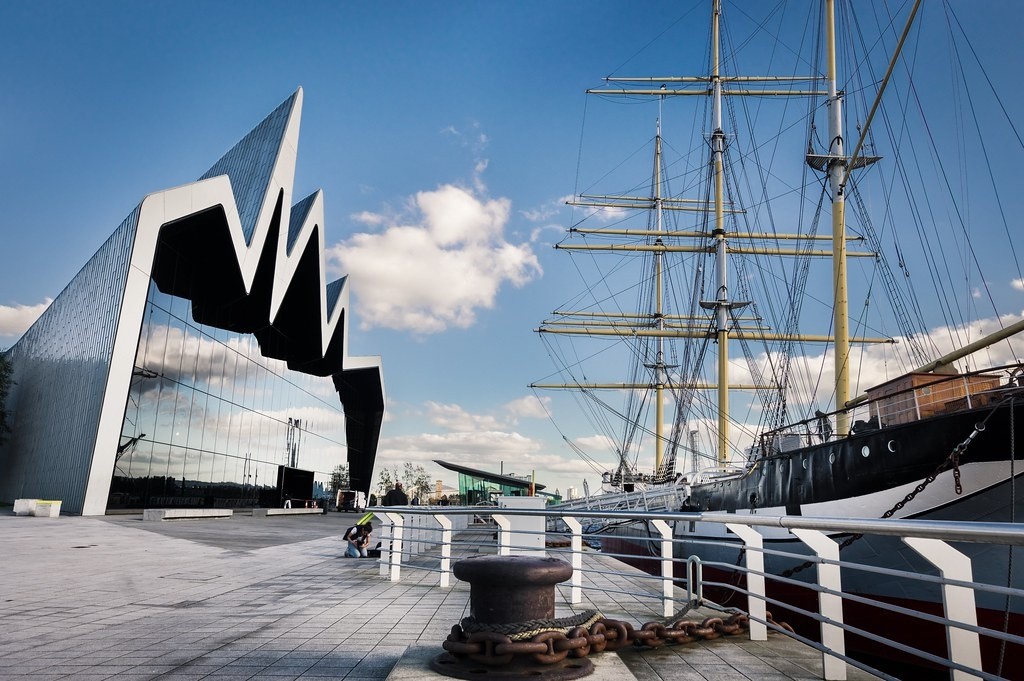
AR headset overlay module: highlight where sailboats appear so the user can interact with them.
[525,0,1024,681]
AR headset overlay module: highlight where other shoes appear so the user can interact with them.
[345,547,350,558]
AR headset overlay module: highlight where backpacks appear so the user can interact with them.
[342,525,367,541]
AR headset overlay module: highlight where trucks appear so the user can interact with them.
[336,490,366,513]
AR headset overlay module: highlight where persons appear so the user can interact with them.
[411,495,420,505]
[343,522,373,558]
[383,482,408,506]
[283,495,291,509]
